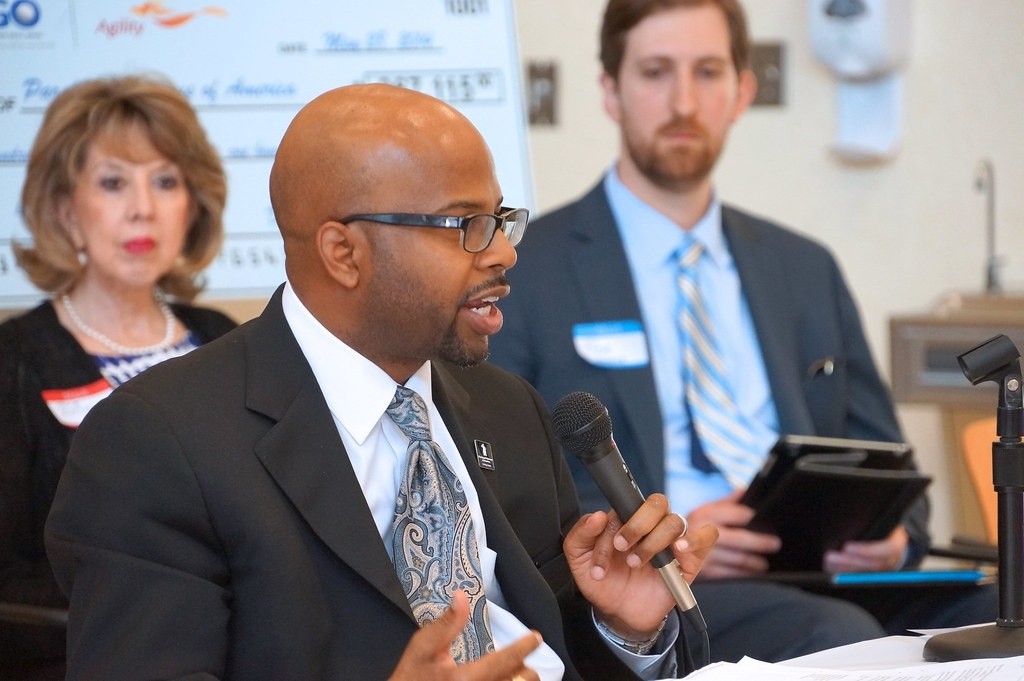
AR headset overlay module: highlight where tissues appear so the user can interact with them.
[805,0,910,160]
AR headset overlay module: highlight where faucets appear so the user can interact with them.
[968,151,1011,290]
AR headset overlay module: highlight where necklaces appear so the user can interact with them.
[63,296,175,356]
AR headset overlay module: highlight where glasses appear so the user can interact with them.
[339,206,529,253]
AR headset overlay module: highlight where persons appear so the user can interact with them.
[485,0,1000,663]
[0,77,239,680]
[46,83,719,681]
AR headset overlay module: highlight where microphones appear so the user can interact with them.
[550,391,707,632]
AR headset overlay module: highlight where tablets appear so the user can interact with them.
[738,434,913,507]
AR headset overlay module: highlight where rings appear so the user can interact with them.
[678,514,689,537]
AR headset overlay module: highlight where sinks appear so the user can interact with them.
[941,295,1022,313]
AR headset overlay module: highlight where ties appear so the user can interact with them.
[673,237,769,490]
[385,386,494,666]
